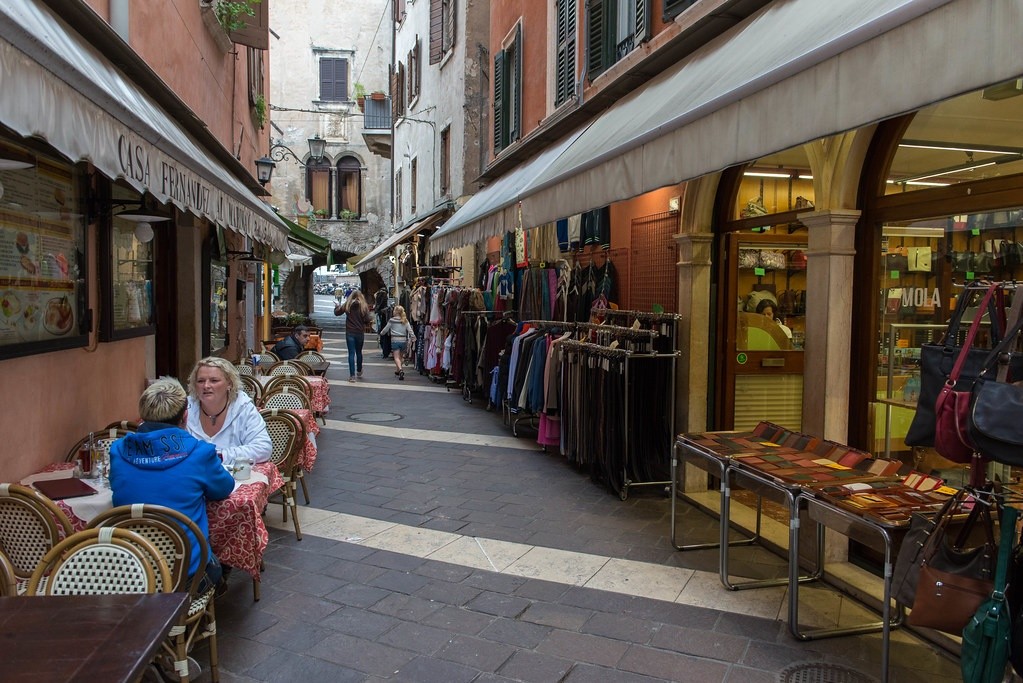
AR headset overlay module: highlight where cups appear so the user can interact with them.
[254,366,261,379]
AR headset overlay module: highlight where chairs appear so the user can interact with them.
[0,330,332,683]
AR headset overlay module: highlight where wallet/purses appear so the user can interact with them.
[681,420,977,529]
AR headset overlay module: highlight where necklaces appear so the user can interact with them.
[200,392,229,425]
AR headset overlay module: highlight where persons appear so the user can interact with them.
[184,357,273,596]
[335,286,352,305]
[756,299,777,321]
[271,326,310,360]
[110,379,236,644]
[334,290,371,382]
[380,306,416,381]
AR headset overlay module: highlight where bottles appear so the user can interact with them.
[252,356,256,367]
[73,432,106,478]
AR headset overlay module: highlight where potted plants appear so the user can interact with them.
[354,81,385,113]
[340,209,356,220]
[315,209,326,219]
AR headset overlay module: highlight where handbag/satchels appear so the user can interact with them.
[889,483,998,611]
[959,506,1017,683]
[737,195,1022,361]
[967,317,1023,468]
[1007,542,1023,678]
[935,282,1012,486]
[906,488,1009,637]
[903,281,1023,447]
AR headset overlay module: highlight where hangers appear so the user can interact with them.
[511,321,576,346]
[582,259,594,292]
[569,260,581,296]
[595,261,615,308]
[557,259,568,296]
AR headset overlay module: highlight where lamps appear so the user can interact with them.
[115,191,172,244]
[744,140,1023,186]
[252,153,277,188]
[270,131,327,172]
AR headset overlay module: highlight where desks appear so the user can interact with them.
[725,449,900,633]
[672,430,821,585]
[14,458,282,604]
[1,592,190,683]
[274,335,325,352]
[792,478,977,682]
[259,361,331,376]
[252,374,329,425]
[252,408,320,508]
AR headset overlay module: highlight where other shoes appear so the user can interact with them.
[399,369,405,380]
[395,370,400,375]
[213,576,228,600]
[356,368,364,379]
[347,375,356,382]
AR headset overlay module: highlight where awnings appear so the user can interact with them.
[0,0,290,253]
[428,0,1022,255]
[354,210,443,273]
[279,213,331,272]
